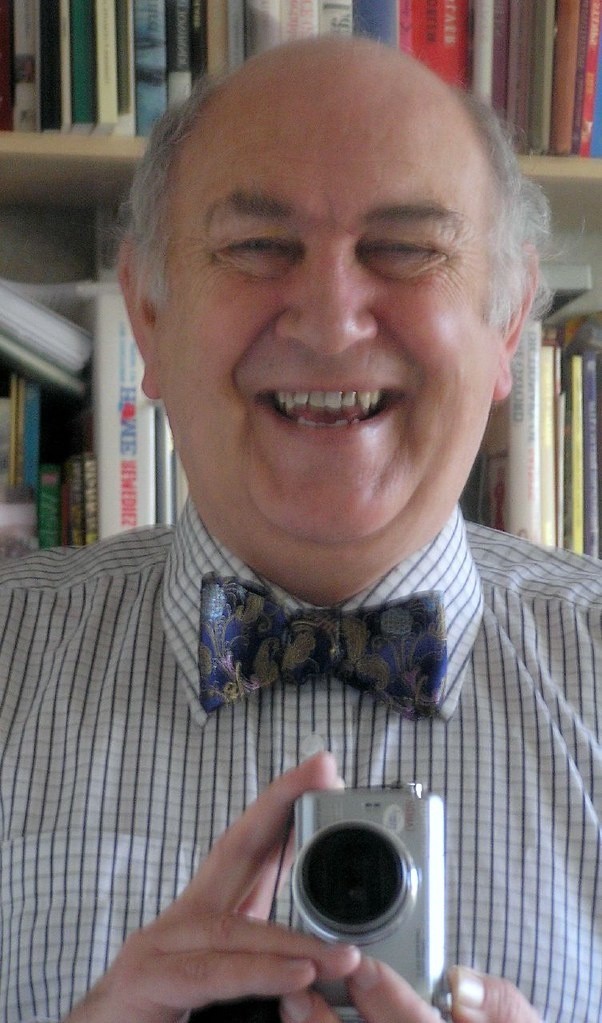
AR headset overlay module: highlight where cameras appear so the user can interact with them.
[293,787,446,1007]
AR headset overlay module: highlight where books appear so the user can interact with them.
[0,202,602,563]
[0,0,602,161]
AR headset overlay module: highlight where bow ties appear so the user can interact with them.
[195,571,447,724]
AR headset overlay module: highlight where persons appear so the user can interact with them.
[0,33,602,1023]
